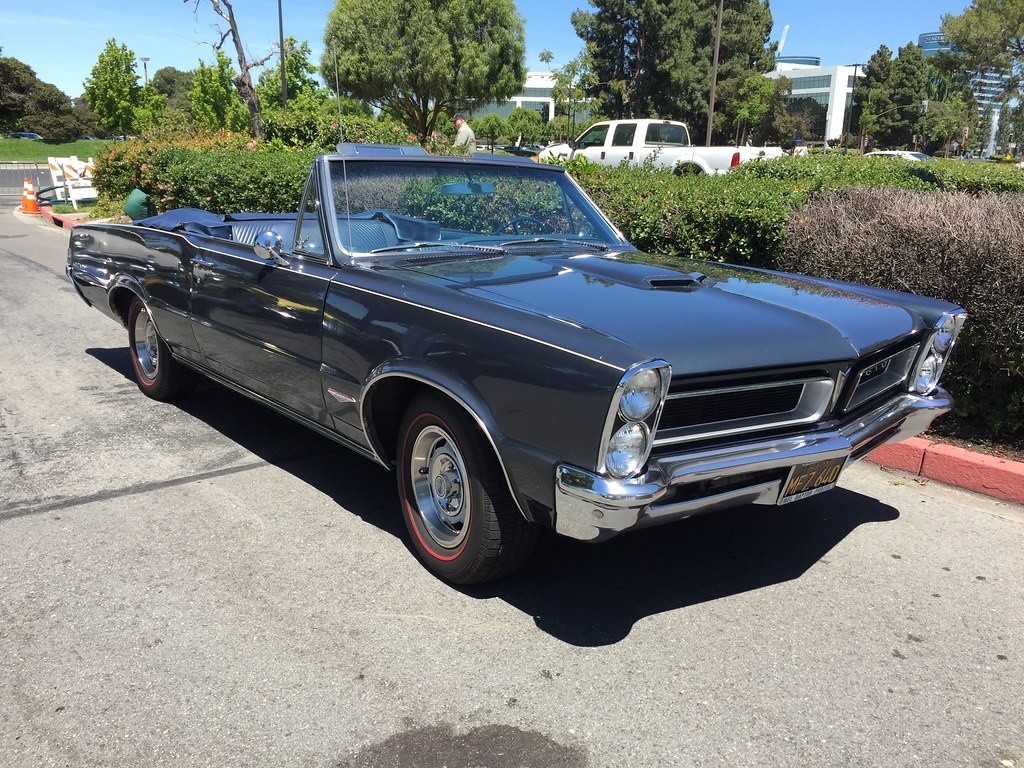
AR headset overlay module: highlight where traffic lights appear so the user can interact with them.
[964,129,967,138]
[961,144,965,148]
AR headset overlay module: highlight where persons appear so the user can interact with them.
[450,114,476,152]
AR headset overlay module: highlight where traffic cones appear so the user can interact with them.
[18,178,41,214]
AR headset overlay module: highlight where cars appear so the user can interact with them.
[865,152,934,161]
[475,143,545,158]
[77,135,100,140]
[66,144,970,590]
[8,132,43,140]
[105,135,124,139]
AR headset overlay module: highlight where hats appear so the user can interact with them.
[450,113,465,122]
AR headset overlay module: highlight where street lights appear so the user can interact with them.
[139,57,150,85]
[844,63,868,153]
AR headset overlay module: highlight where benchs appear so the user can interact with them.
[222,218,401,258]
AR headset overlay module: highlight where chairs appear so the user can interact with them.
[615,133,628,145]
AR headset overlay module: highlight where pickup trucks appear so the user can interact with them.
[539,118,784,175]
